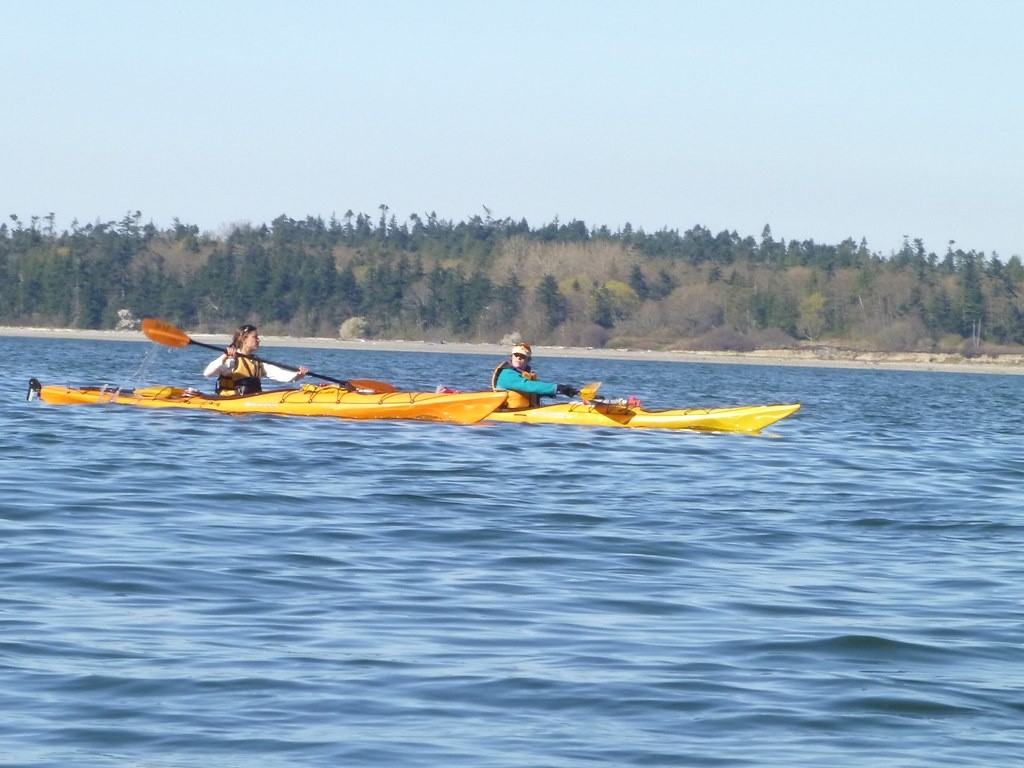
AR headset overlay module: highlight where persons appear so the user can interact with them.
[204,324,309,396]
[492,341,579,409]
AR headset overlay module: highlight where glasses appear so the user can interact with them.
[512,353,527,359]
[244,326,253,331]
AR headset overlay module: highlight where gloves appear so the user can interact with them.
[557,384,578,398]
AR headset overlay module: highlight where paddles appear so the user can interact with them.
[141,318,398,393]
[557,381,602,400]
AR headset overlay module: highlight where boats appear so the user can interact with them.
[28,378,507,428]
[435,385,802,436]
